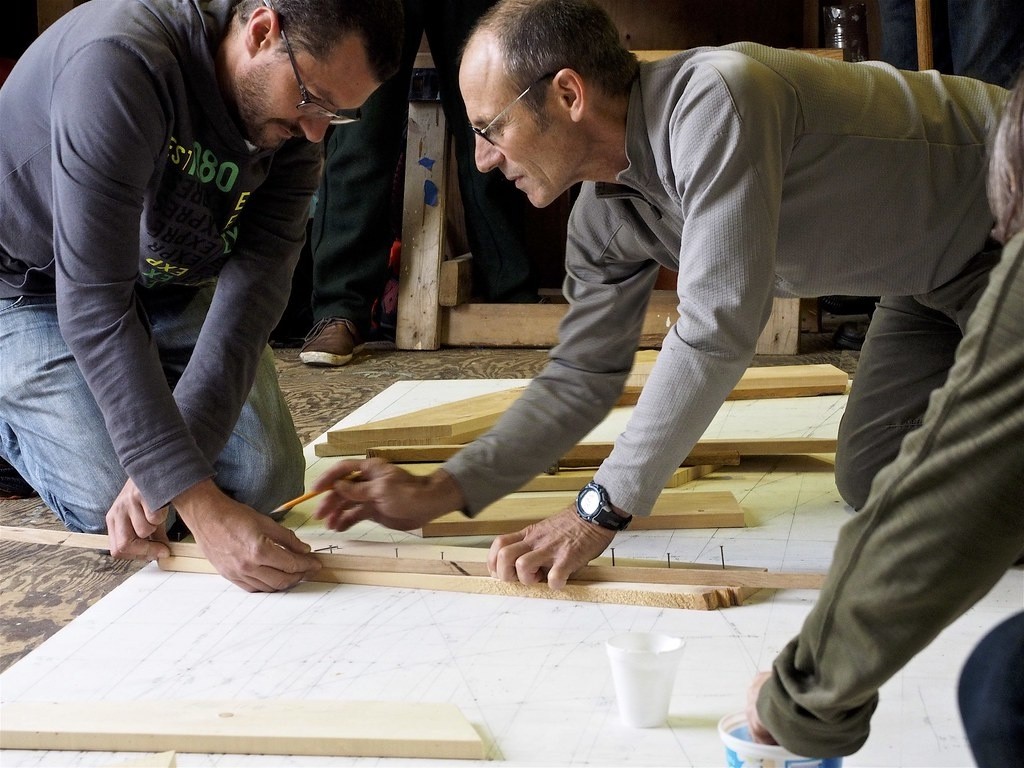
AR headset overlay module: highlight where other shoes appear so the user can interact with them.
[1,459,34,500]
[834,322,868,351]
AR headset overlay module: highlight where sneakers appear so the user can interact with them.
[295,316,366,364]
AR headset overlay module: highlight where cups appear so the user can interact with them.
[716,711,843,768]
[605,631,687,728]
[822,0,868,63]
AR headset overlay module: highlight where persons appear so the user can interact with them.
[297,0,565,366]
[748,88,1024,768]
[311,0,1024,588]
[877,0,1024,85]
[0,0,421,597]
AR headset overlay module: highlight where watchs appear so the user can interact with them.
[576,483,632,531]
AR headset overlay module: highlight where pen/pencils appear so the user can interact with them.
[269,470,364,515]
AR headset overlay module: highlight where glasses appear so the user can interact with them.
[470,72,558,146]
[262,1,361,124]
